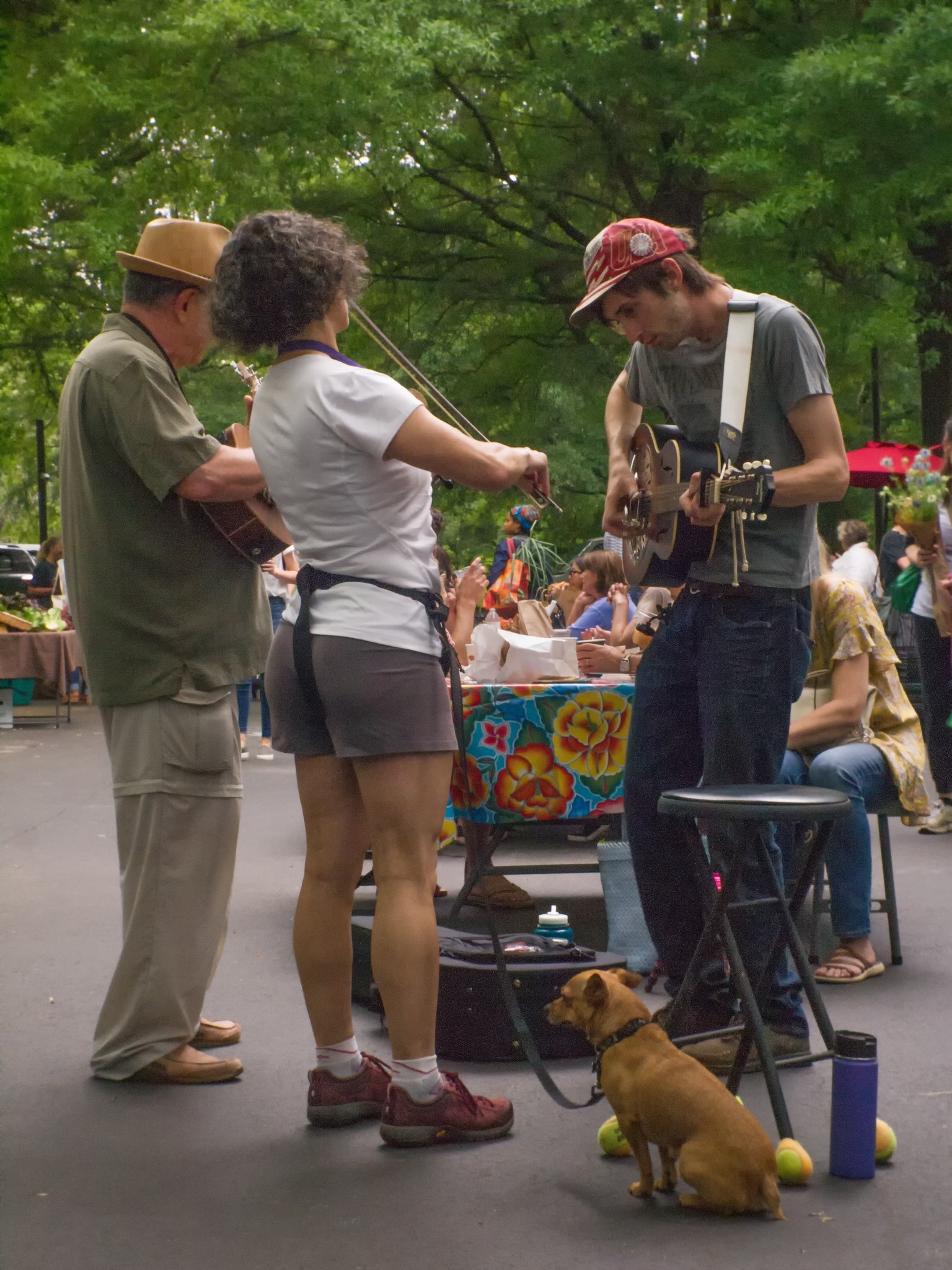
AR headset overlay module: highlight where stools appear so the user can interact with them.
[808,803,914,967]
[656,784,853,1140]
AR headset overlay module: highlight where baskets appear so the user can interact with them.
[0,612,32,631]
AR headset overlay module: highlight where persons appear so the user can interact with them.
[772,532,931,983]
[59,606,92,704]
[27,535,63,610]
[430,504,551,908]
[236,543,300,760]
[829,418,952,835]
[543,549,685,842]
[209,210,548,1147]
[56,220,265,1087]
[569,218,850,1078]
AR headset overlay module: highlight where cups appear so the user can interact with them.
[576,639,606,674]
[465,644,476,665]
[632,609,660,649]
[454,563,482,581]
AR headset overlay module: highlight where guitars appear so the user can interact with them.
[197,361,294,567]
[622,423,777,588]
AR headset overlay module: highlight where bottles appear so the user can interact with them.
[533,904,574,947]
[484,608,501,629]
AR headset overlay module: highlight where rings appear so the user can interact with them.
[587,659,589,666]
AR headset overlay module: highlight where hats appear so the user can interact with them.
[115,218,231,286]
[511,504,540,531]
[570,217,687,325]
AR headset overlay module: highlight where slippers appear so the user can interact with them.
[435,884,447,897]
[814,945,884,982]
[463,883,535,908]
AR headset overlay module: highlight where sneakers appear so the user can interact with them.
[379,1070,514,1145]
[306,1052,392,1126]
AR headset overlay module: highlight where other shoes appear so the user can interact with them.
[61,690,80,704]
[240,749,249,759]
[681,1026,812,1075]
[256,748,274,760]
[128,1044,243,1083]
[187,1019,241,1048]
[85,695,92,706]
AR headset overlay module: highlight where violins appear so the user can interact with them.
[406,388,454,490]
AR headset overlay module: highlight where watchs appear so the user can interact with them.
[619,654,631,673]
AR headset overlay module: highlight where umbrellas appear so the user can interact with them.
[844,439,946,531]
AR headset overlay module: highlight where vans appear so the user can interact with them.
[0,543,67,610]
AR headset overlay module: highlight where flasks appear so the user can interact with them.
[829,1029,880,1180]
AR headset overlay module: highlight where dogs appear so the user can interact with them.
[541,968,785,1222]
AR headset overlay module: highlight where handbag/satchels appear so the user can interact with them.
[462,624,579,683]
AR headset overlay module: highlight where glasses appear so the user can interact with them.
[569,569,582,575]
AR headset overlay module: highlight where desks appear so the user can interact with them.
[437,673,635,920]
[0,629,88,728]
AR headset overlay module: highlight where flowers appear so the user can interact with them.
[879,447,952,523]
